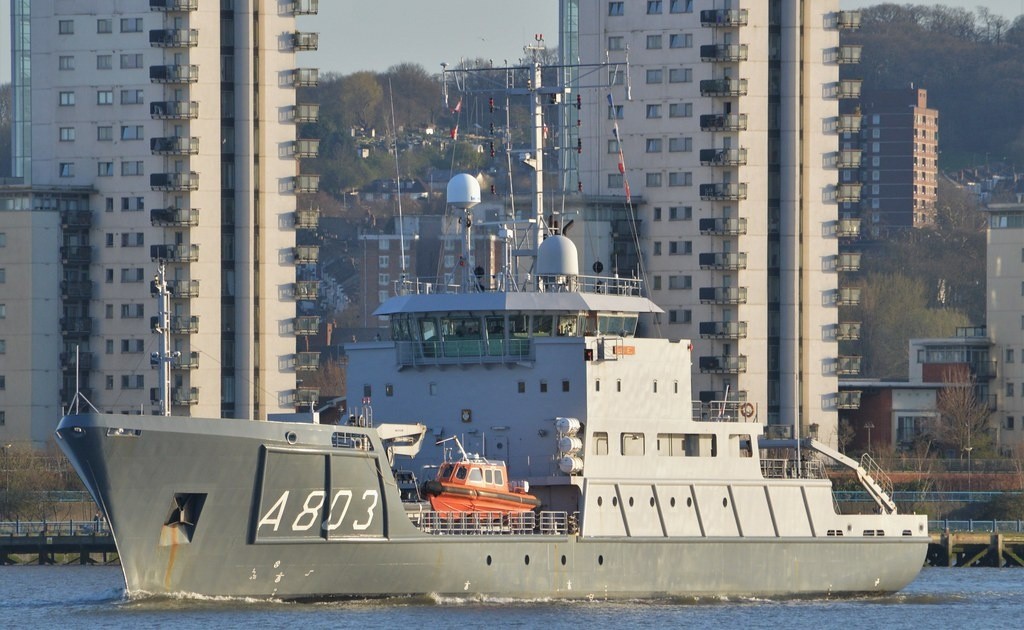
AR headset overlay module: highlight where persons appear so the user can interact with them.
[455,320,469,333]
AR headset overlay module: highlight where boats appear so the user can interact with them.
[57,37,932,605]
[422,455,540,525]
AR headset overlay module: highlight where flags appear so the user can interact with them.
[450,98,462,140]
[607,94,631,202]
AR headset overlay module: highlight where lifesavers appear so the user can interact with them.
[740,402,754,417]
